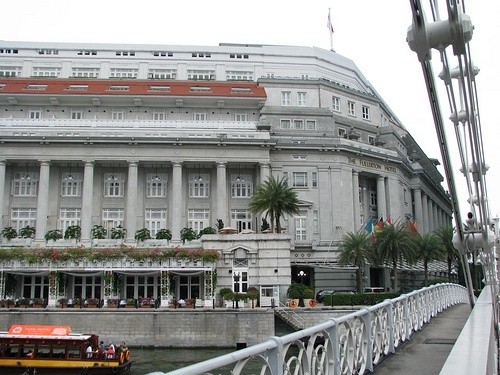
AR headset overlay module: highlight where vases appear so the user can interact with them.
[142,304,150,308]
[223,299,245,308]
[88,304,97,308]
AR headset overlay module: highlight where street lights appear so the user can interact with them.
[297,270,306,307]
[234,270,241,308]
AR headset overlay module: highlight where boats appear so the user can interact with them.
[0,324,134,375]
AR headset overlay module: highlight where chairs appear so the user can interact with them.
[194,298,215,310]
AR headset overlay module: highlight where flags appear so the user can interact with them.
[365,215,402,241]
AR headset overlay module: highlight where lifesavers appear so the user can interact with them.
[290,300,297,307]
[309,300,316,307]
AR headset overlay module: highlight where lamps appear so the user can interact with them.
[21,160,32,180]
[107,162,119,182]
[194,163,204,183]
[235,163,245,183]
[64,161,74,182]
[151,161,160,182]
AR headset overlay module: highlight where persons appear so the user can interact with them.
[134,297,160,309]
[26,350,33,358]
[86,340,128,359]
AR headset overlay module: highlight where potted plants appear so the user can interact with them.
[0,297,46,308]
[72,296,85,307]
[125,298,136,308]
[0,248,219,268]
[288,284,299,307]
[303,289,316,307]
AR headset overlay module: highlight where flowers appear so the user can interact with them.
[140,297,153,306]
[87,296,97,304]
